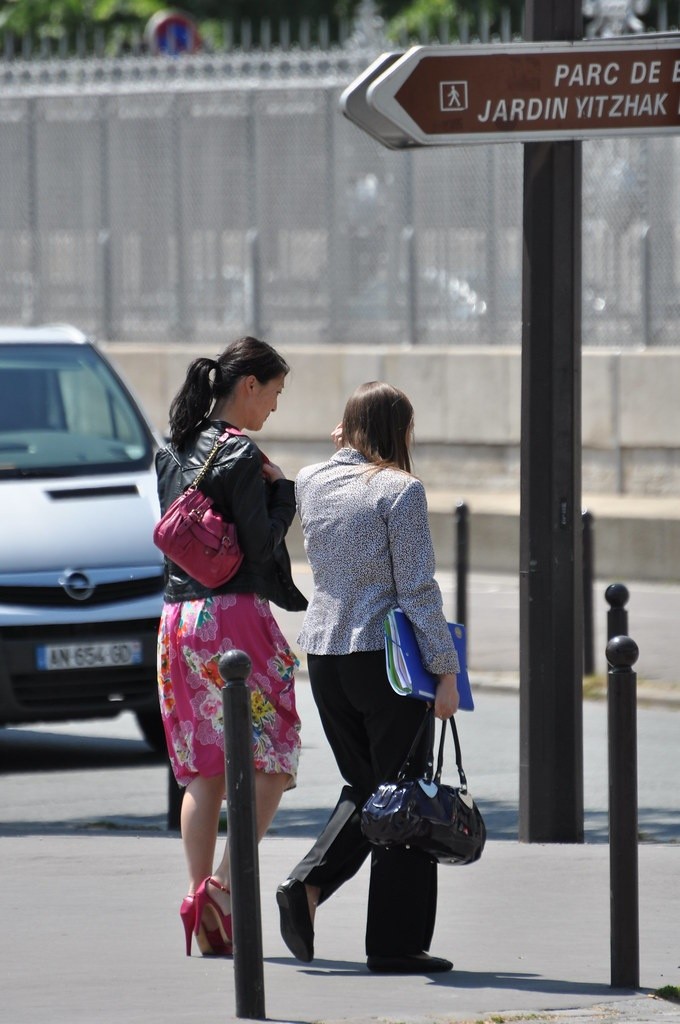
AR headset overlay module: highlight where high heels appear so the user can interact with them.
[180,894,232,956]
[194,877,233,944]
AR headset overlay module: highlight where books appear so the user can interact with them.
[381,608,477,713]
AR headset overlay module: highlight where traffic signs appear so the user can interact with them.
[364,37,680,145]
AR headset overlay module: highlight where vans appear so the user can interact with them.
[0,325,170,750]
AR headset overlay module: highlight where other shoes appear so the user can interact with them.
[276,877,315,962]
[366,952,453,973]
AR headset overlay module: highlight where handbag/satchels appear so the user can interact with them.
[359,706,487,866]
[153,427,270,589]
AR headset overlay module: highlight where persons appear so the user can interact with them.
[154,335,308,956]
[277,380,460,974]
